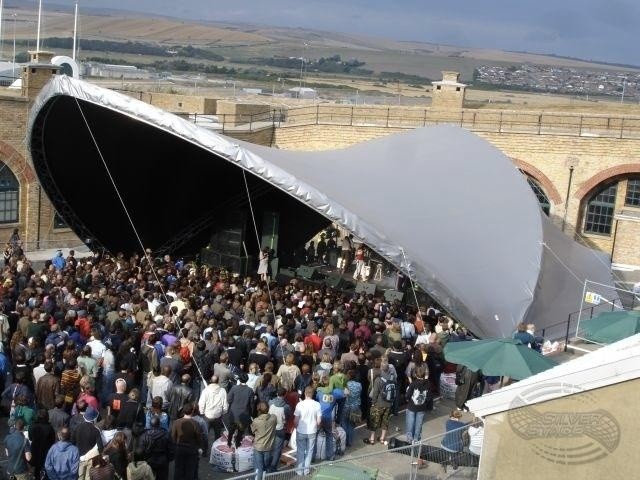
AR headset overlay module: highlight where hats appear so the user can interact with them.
[394,341,402,349]
[83,406,100,423]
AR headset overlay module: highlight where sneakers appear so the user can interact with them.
[363,438,375,445]
[377,437,385,445]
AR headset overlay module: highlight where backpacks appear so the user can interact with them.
[379,373,398,403]
[393,359,405,380]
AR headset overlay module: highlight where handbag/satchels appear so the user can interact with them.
[348,409,362,423]
[132,422,144,436]
[462,430,469,446]
[39,469,47,479]
[181,347,190,363]
[368,387,373,399]
[455,373,464,385]
[275,430,285,437]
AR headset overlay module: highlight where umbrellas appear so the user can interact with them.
[443,337,560,388]
[578,309,640,346]
